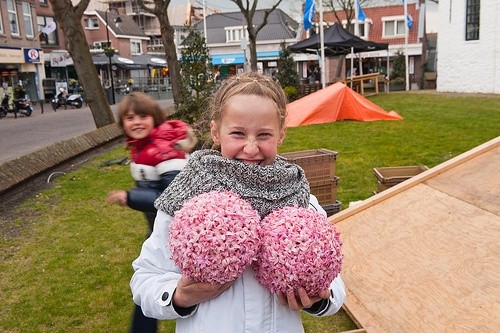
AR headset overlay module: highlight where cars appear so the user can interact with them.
[116,84,130,94]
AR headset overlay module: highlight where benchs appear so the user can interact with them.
[296,82,335,99]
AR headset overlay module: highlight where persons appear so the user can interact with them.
[130,72,347,333]
[106,92,199,333]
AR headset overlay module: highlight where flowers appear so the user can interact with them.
[251,207,344,299]
[166,185,261,282]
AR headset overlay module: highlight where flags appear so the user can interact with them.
[304,0,316,30]
[355,0,367,22]
[402,0,413,29]
[43,21,57,36]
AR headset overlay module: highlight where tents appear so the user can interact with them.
[66,52,186,107]
[285,82,404,128]
[286,23,390,95]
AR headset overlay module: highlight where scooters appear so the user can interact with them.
[0,92,33,118]
[51,88,83,109]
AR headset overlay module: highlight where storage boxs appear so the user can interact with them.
[309,176,340,204]
[279,148,338,183]
[373,166,430,195]
[319,200,342,218]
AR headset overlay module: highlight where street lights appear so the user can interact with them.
[105,7,123,104]
[317,19,330,33]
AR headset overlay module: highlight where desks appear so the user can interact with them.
[346,73,380,98]
